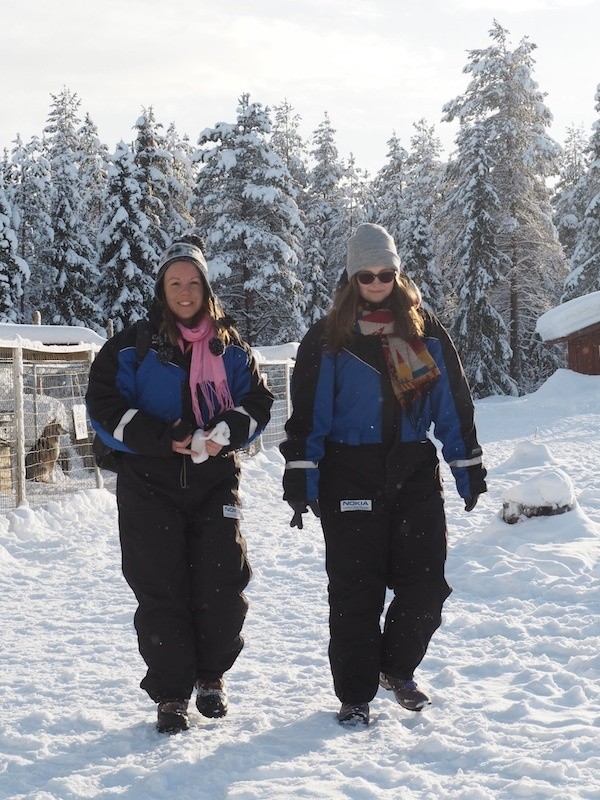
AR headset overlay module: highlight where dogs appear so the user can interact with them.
[25,419,69,483]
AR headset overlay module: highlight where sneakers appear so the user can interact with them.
[337,701,369,725]
[194,677,228,718]
[378,669,430,711]
[154,694,191,736]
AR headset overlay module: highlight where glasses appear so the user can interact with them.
[356,269,396,285]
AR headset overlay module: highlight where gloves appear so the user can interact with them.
[464,494,478,511]
[287,499,320,529]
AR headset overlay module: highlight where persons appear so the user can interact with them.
[85,235,273,738]
[279,222,488,727]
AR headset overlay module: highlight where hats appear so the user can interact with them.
[346,224,401,281]
[154,236,224,357]
